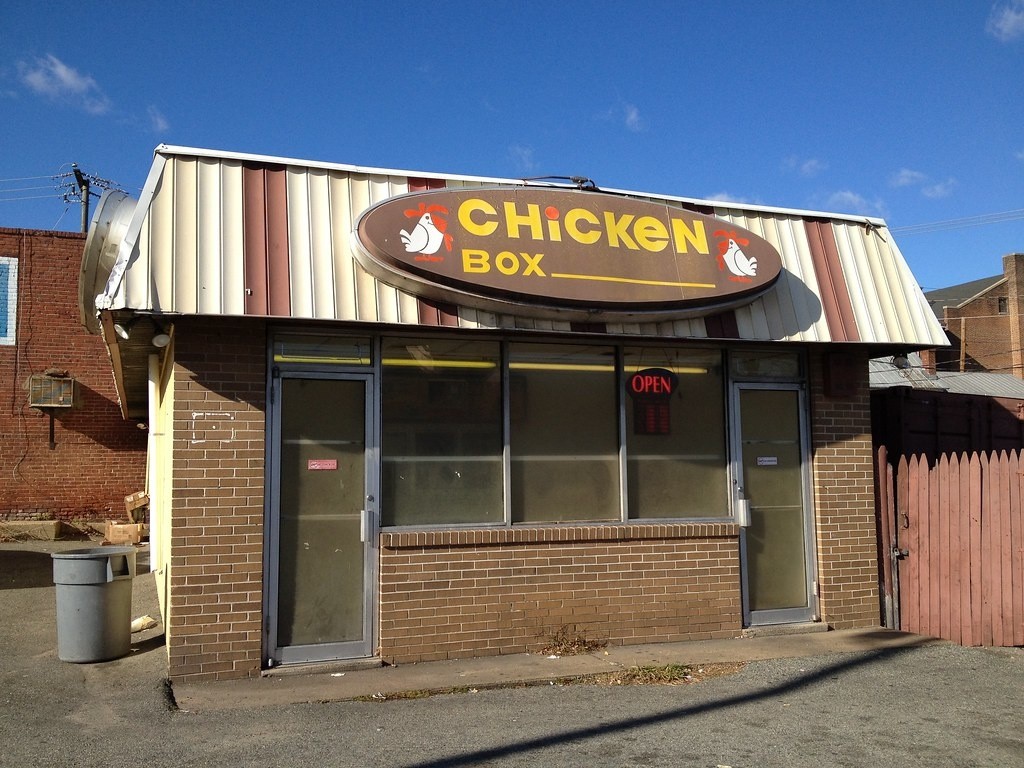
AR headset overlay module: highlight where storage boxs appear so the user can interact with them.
[103,490,149,544]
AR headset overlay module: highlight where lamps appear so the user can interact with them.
[893,348,907,367]
[153,319,171,348]
[112,315,143,339]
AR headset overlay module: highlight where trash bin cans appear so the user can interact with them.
[51,546,135,664]
